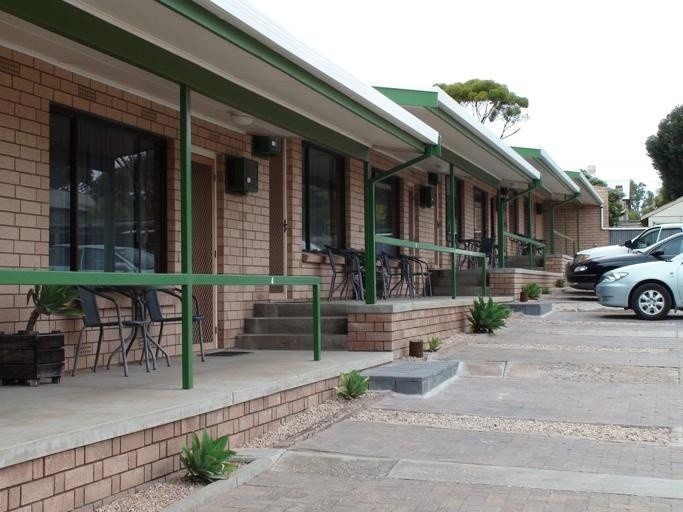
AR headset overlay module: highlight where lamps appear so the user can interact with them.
[225,110,255,127]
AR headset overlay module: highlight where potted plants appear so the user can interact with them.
[0,283,88,387]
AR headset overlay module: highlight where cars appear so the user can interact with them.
[48,244,155,274]
[565,222,681,319]
[376,233,393,254]
[301,239,317,251]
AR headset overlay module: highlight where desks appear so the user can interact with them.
[92,284,174,371]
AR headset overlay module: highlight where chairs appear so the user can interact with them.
[125,285,205,374]
[323,238,498,303]
[70,285,151,377]
